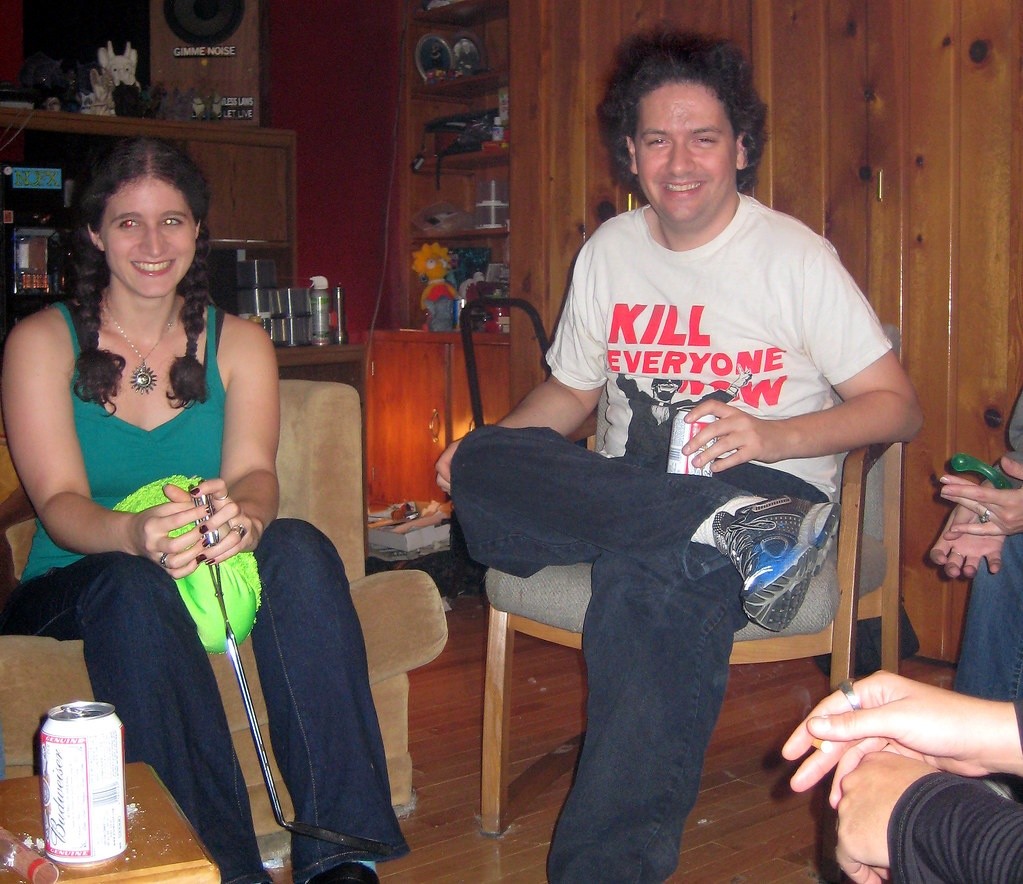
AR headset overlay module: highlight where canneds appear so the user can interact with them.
[666,409,717,478]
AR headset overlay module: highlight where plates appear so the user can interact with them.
[415,33,453,79]
[451,31,486,73]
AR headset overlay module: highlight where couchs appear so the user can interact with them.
[0,380,448,865]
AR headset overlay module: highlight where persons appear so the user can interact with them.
[781,669,1023,884]
[0,137,411,884]
[929,387,1023,704]
[437,27,924,884]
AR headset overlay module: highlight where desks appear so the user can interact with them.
[0,763,223,884]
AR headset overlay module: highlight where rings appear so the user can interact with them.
[230,524,246,540]
[214,491,229,501]
[837,677,861,710]
[979,509,991,524]
[160,553,168,567]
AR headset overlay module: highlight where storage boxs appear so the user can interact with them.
[368,524,451,552]
[425,131,459,156]
[498,84,510,121]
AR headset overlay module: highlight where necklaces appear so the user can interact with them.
[105,283,179,396]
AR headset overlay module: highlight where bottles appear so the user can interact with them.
[493,117,504,141]
[486,289,509,333]
[18,271,49,294]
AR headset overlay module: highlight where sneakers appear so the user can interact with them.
[712,495,840,631]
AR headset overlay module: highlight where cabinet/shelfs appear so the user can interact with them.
[349,0,510,511]
[0,107,364,454]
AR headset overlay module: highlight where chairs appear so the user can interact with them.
[482,325,905,834]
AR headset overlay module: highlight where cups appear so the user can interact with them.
[270,286,311,346]
